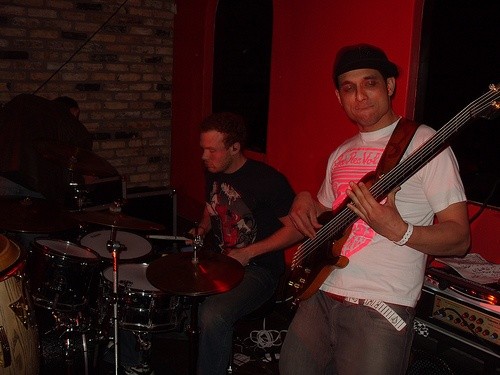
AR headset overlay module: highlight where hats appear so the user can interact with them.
[332,43,400,89]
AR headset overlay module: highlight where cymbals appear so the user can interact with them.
[63,209,166,230]
[145,250,245,300]
[0,192,82,234]
[25,140,118,178]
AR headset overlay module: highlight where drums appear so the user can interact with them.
[27,236,101,309]
[76,228,156,317]
[96,262,202,375]
[33,305,101,375]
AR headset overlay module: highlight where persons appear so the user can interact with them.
[102,113,296,375]
[279,44,470,375]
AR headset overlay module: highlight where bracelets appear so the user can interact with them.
[393,222,414,246]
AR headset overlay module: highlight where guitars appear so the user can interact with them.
[270,81,500,311]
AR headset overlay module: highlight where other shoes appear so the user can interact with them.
[108,360,155,375]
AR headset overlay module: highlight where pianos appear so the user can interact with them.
[412,265,500,359]
